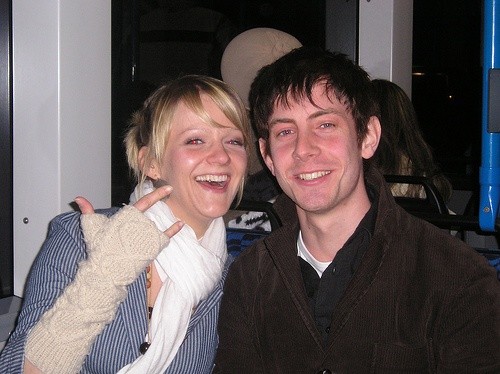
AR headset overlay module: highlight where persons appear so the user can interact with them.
[0,74,253,374]
[212,48,500,374]
[219,27,458,242]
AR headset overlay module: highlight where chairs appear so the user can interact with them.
[223,200,283,256]
[383,174,449,216]
[421,216,500,279]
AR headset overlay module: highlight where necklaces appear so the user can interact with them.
[139,265,202,354]
